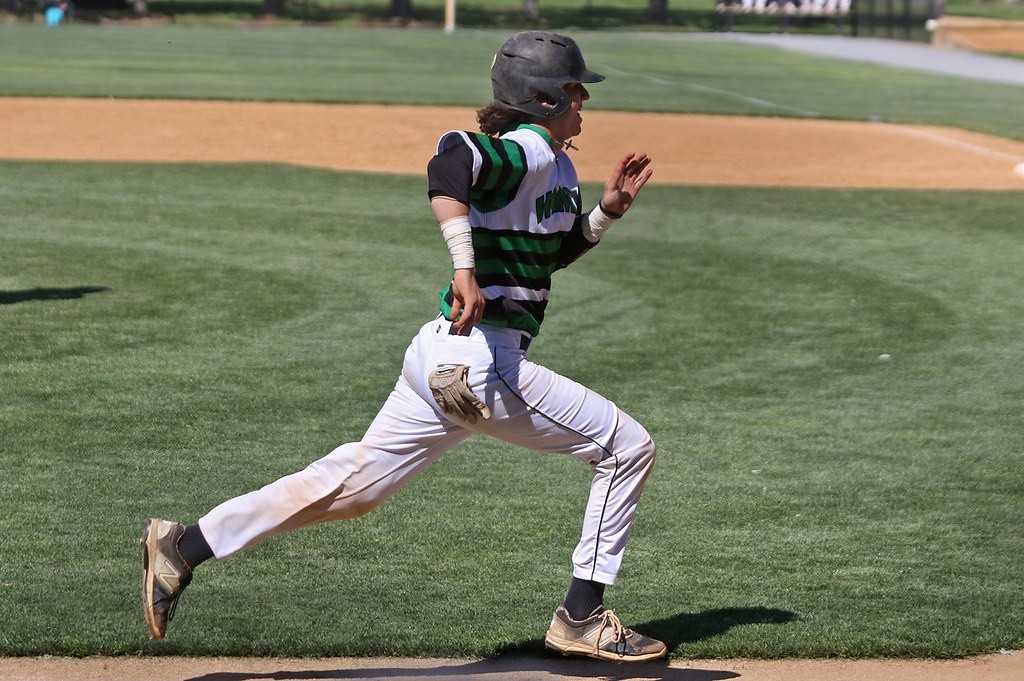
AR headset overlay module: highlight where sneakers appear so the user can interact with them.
[140,517,194,641]
[544,603,667,662]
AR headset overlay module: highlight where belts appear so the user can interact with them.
[445,322,533,350]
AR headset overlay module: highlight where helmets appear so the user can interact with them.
[489,30,606,120]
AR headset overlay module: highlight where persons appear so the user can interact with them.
[136,35,665,664]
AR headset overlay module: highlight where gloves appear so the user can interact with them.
[428,366,491,424]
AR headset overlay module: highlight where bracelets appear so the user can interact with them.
[599,200,623,218]
